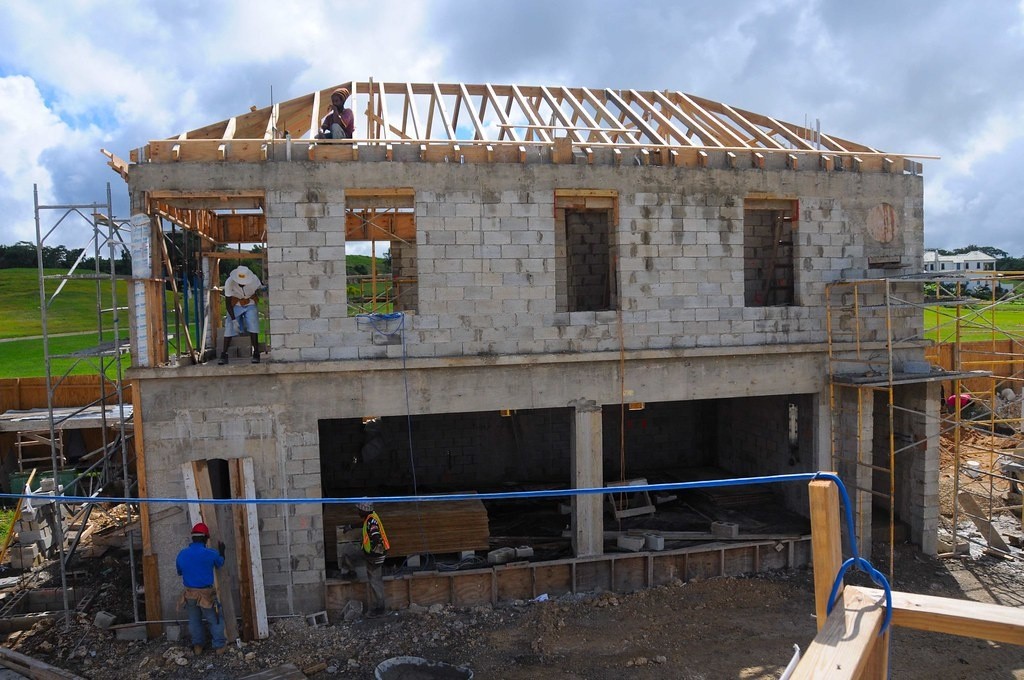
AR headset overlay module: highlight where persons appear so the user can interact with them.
[999,389,1016,404]
[218,266,262,365]
[941,395,975,419]
[336,502,390,620]
[314,88,355,145]
[175,522,226,655]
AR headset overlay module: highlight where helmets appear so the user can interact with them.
[191,522,211,539]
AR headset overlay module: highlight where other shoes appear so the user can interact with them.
[251,351,261,364]
[367,610,385,619]
[216,642,227,655]
[193,644,204,656]
[217,352,229,365]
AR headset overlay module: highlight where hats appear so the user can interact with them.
[354,495,377,514]
[331,87,350,104]
[230,264,255,286]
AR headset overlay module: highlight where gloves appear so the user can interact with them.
[232,319,241,334]
[217,540,226,558]
